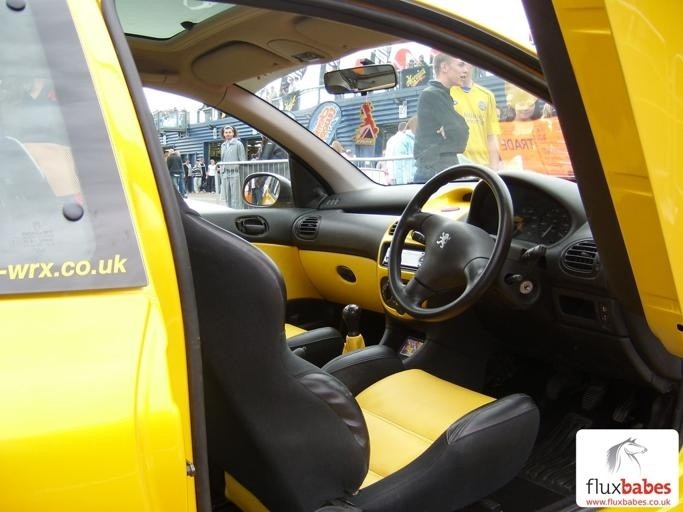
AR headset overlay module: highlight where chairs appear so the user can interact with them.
[2,138,540,511]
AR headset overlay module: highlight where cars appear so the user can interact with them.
[0,1,682,512]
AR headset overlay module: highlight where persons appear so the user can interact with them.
[412,50,469,182]
[449,62,501,173]
[161,147,220,199]
[219,124,247,210]
[254,135,291,182]
[499,89,543,122]
[493,104,502,122]
[384,120,406,186]
[395,116,418,184]
[331,140,351,159]
